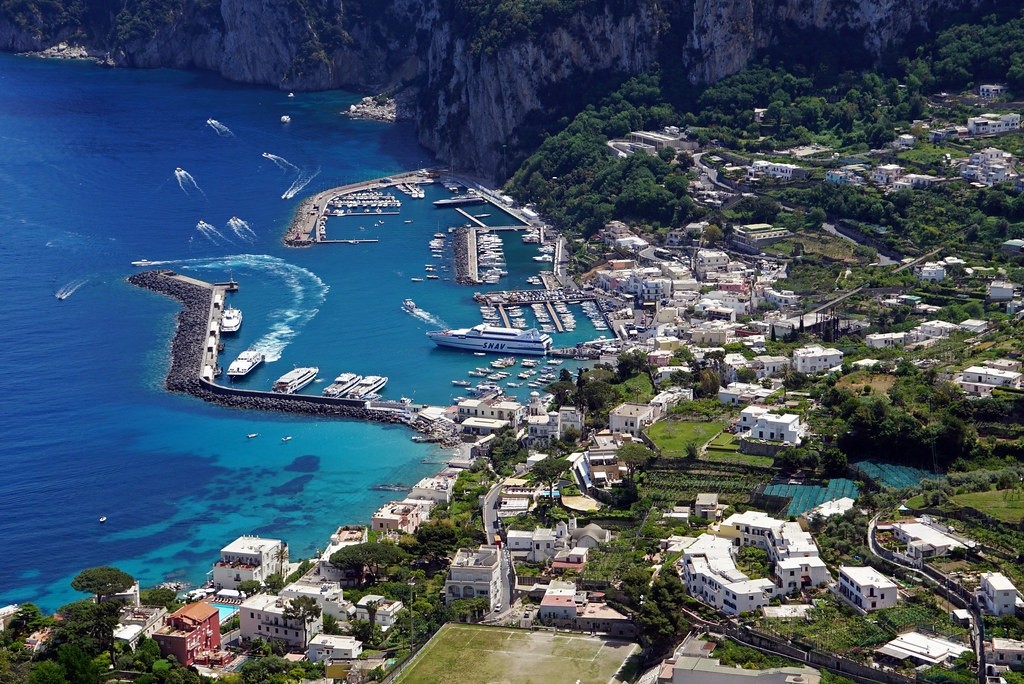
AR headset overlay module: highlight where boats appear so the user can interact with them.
[322,374,362,399]
[220,308,243,334]
[466,357,590,397]
[451,379,472,387]
[479,301,608,332]
[426,324,553,357]
[226,351,265,378]
[273,365,319,393]
[477,232,555,285]
[321,174,452,282]
[403,298,416,313]
[346,375,389,400]
